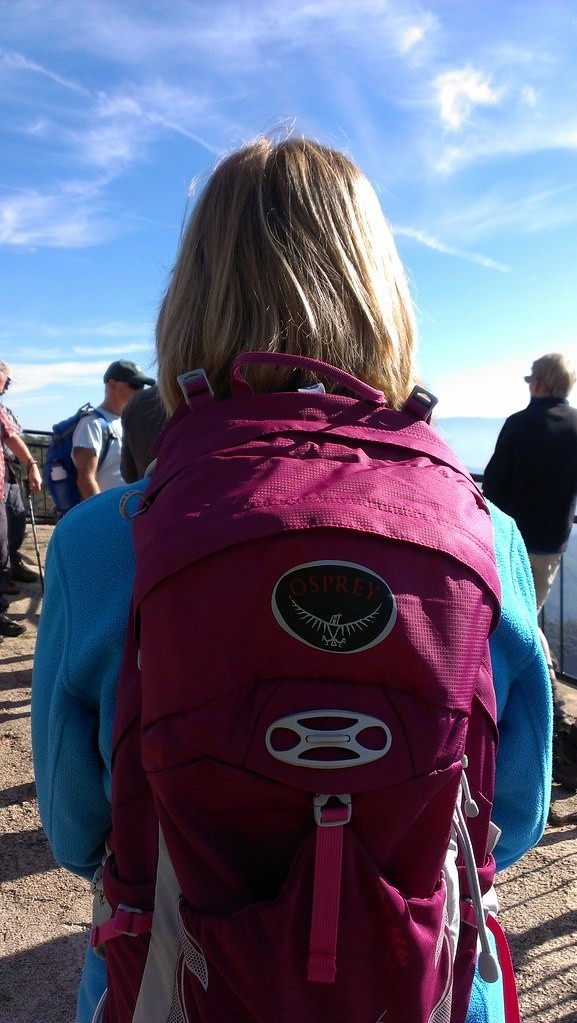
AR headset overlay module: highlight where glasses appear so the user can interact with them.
[524,376,534,383]
[123,381,146,389]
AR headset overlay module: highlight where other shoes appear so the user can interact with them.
[0,615,26,636]
[11,561,38,582]
[0,575,21,595]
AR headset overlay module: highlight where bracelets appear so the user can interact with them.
[27,457,38,473]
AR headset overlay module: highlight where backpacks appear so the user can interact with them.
[91,350,521,1023]
[43,403,111,519]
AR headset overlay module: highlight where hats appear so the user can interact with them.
[104,359,156,386]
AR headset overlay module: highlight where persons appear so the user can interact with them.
[31,141,553,1023]
[70,360,155,499]
[481,353,577,708]
[0,361,42,643]
